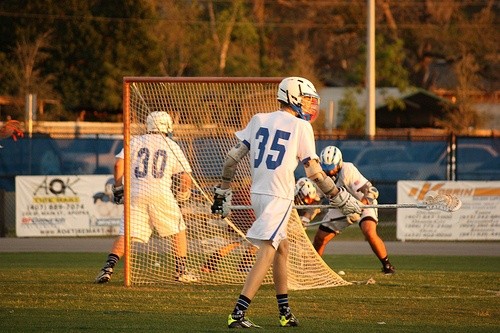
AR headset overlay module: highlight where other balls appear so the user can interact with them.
[153,262,161,268]
[338,270,345,276]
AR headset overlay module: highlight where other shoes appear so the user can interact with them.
[383,265,395,275]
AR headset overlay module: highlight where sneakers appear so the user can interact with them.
[227,313,264,330]
[279,314,299,327]
[95,268,113,283]
[175,275,197,283]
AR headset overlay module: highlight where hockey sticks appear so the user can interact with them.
[197,215,348,255]
[218,192,463,211]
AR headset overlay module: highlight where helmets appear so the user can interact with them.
[146,111,173,135]
[277,77,320,109]
[320,145,342,171]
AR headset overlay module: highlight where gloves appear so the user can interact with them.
[210,185,233,219]
[329,186,365,215]
[111,185,125,204]
[346,213,361,225]
[177,190,191,208]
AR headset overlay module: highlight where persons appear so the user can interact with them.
[94,111,199,283]
[197,176,326,273]
[302,144,395,273]
[211,76,364,328]
